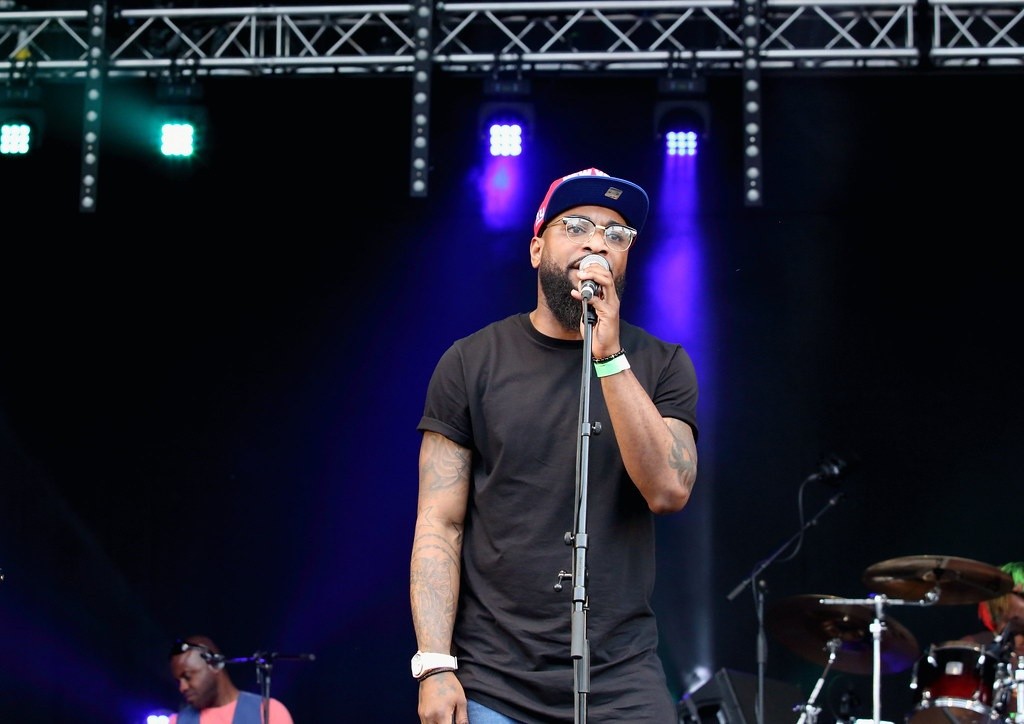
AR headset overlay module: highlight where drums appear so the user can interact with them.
[903,640,1014,723]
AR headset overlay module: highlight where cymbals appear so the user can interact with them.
[764,594,916,675]
[866,557,1015,605]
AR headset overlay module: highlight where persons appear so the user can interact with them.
[411,167,700,724]
[927,563,1024,724]
[167,636,293,724]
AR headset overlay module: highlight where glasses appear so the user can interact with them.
[547,214,637,252]
[169,641,215,657]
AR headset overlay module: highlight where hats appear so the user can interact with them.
[533,168,650,236]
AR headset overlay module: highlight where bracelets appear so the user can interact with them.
[592,348,625,363]
[417,668,454,682]
[594,355,630,377]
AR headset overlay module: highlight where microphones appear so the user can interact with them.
[807,461,846,486]
[200,652,224,672]
[989,619,1012,656]
[579,254,613,302]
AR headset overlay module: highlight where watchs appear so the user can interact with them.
[411,650,458,678]
